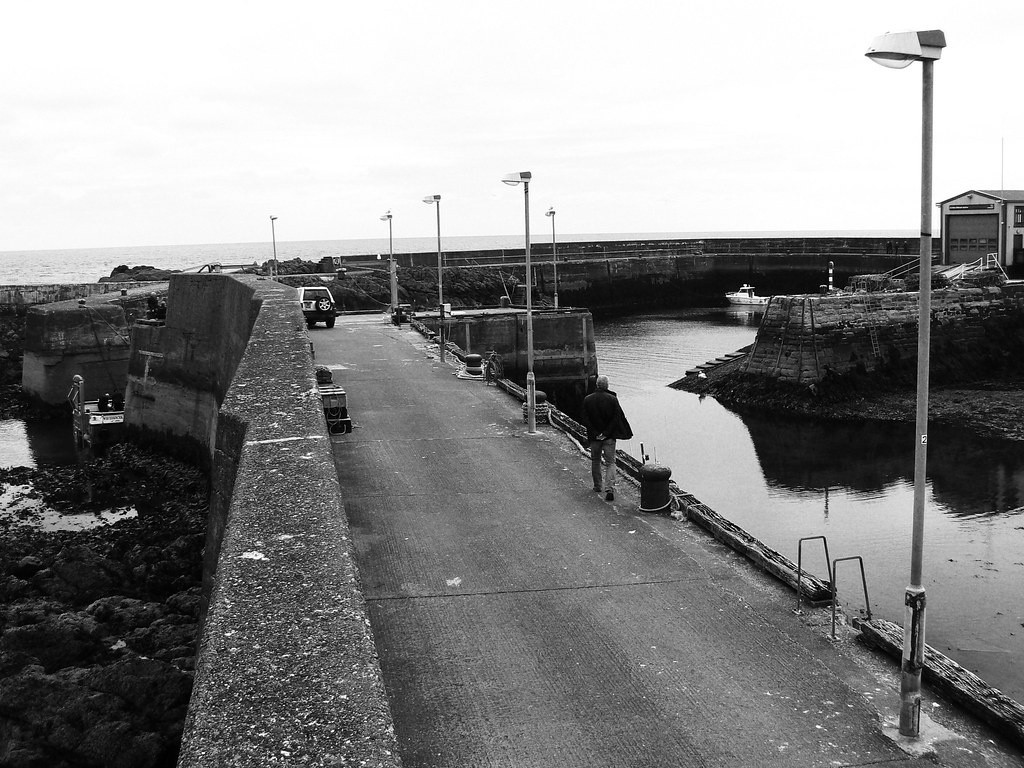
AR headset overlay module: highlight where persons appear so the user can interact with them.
[98,392,111,412]
[904,241,908,252]
[895,242,899,254]
[148,292,159,312]
[111,389,124,411]
[886,240,893,254]
[584,375,633,500]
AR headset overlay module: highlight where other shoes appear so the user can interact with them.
[592,487,603,492]
[605,491,614,501]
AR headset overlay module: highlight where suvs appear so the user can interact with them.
[297,286,336,328]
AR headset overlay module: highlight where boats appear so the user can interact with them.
[725,285,771,304]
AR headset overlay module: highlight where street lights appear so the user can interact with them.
[379,212,398,312]
[864,29,946,736]
[502,170,536,434]
[543,205,558,309]
[422,195,444,345]
[270,215,278,281]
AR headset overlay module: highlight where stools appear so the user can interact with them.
[521,391,548,424]
[500,296,509,307]
[465,354,482,375]
[640,464,672,511]
